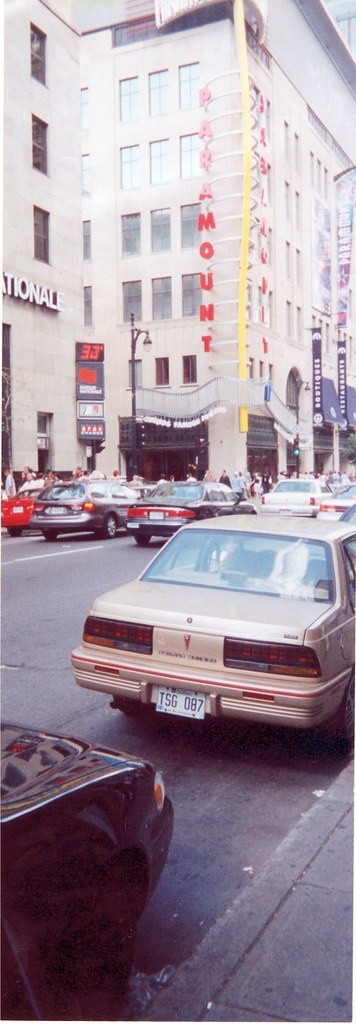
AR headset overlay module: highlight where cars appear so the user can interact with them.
[73,514,356,754]
[128,479,256,547]
[0,716,178,1021]
[31,482,138,542]
[260,478,332,519]
[316,483,356,521]
[2,489,48,537]
[336,503,356,523]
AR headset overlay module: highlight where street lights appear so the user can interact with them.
[127,312,152,481]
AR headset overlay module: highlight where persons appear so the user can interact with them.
[2,465,17,500]
[233,469,356,499]
[202,468,218,482]
[155,474,168,486]
[19,465,88,487]
[185,473,198,483]
[169,475,177,484]
[110,469,122,482]
[219,468,232,489]
[120,469,143,488]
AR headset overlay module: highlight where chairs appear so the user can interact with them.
[228,543,327,590]
